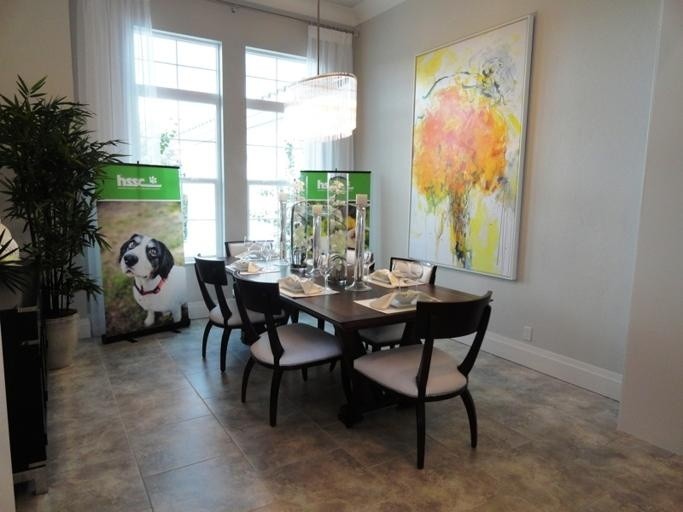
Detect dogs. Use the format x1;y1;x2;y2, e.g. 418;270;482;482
117;234;188;327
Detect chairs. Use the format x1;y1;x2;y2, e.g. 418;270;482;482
351;291;494;471
194;253;287;371
225;241;299;323
233;270;350;428
359;258;437;354
317;248;372;330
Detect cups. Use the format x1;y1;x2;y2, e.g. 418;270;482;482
279;264;288;278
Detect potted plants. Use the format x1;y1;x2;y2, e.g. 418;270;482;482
0;74;132;368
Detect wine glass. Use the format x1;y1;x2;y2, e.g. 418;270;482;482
393;260;424;299
261;243;274;272
244;235;254;252
316;256;335;293
362;251;375;278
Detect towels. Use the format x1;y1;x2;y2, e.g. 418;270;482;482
367;269;398;285
369;291;437;309
276;275;314;294
229;261;261;273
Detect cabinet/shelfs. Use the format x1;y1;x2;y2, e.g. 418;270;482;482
0;312;48;473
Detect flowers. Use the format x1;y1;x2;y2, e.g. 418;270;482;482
292;178;305;246
329;181;345;255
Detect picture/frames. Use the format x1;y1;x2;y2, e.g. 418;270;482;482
408;14;535;282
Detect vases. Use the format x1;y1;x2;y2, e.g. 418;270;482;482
289;175;308;270
327;173;348;285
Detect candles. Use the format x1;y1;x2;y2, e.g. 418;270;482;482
356;194;367;205
312;205;322;216
278;192;288;201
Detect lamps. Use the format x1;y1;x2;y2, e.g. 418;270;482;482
285;0;357;142
